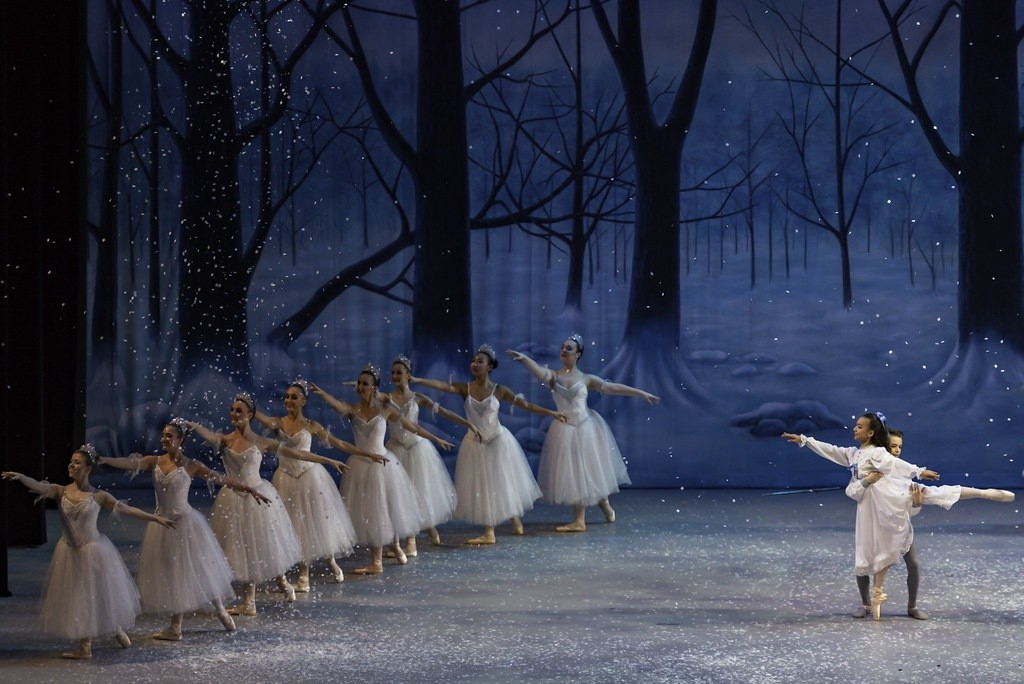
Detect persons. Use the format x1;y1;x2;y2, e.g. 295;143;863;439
343;354;483;545
1;443;176;659
507;333;661;531
781;412;1015;622
252;376;390;592
845;428;928;620
183;390;350;616
308;362;456;575
409;344;569;543
96;416;272;641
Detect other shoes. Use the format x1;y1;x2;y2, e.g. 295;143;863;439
467;534;496;544
512;516;524;535
387;548;405;558
872;602;880;621
853;605;871;617
332;563;344;583
114;625;130;649
215;606;235;631
389;542;408;565
277;579;297;603
62;647;92;659
428;527;440;545
605;509;615;524
986;488;1015;502
908;608;929;620
354;567;383;574
225;603;256;615
404;539;418;556
556;524;587;531
279;576;310;593
153;630;183;641
